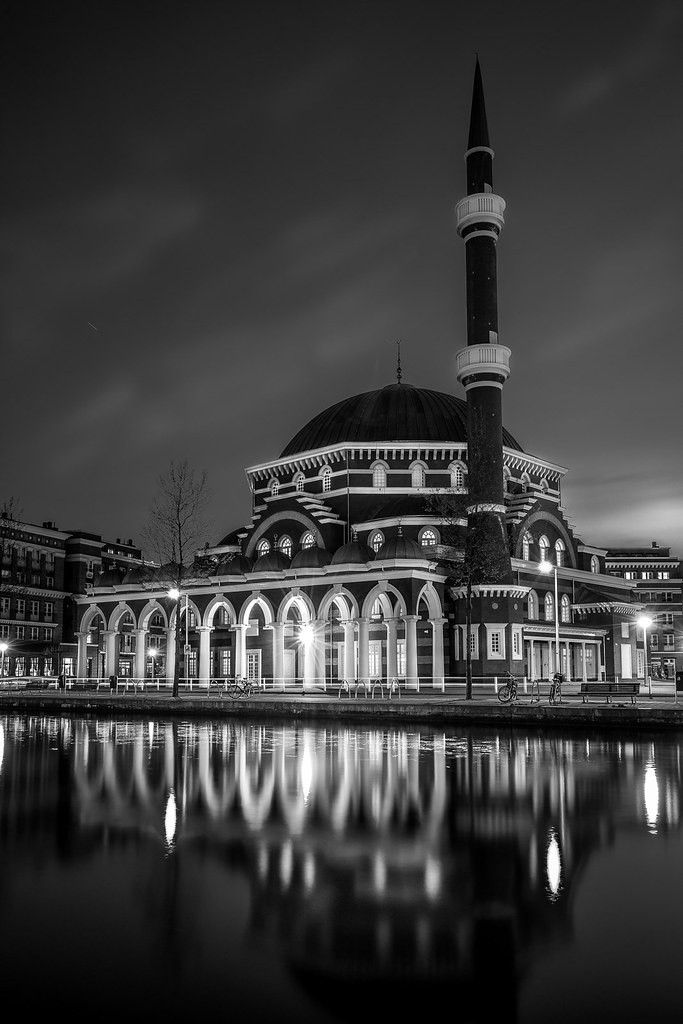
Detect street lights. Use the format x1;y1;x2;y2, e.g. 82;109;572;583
0;643;9;677
168;588;191;691
539;560;563;682
147;649;157;683
638;616;653;686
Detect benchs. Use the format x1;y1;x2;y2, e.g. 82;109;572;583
26;682;49;693
73;683;97;692
577;683;640;704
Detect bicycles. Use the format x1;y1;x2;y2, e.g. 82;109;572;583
548;672;561;706
227;674;255;700
497;671;520;702
